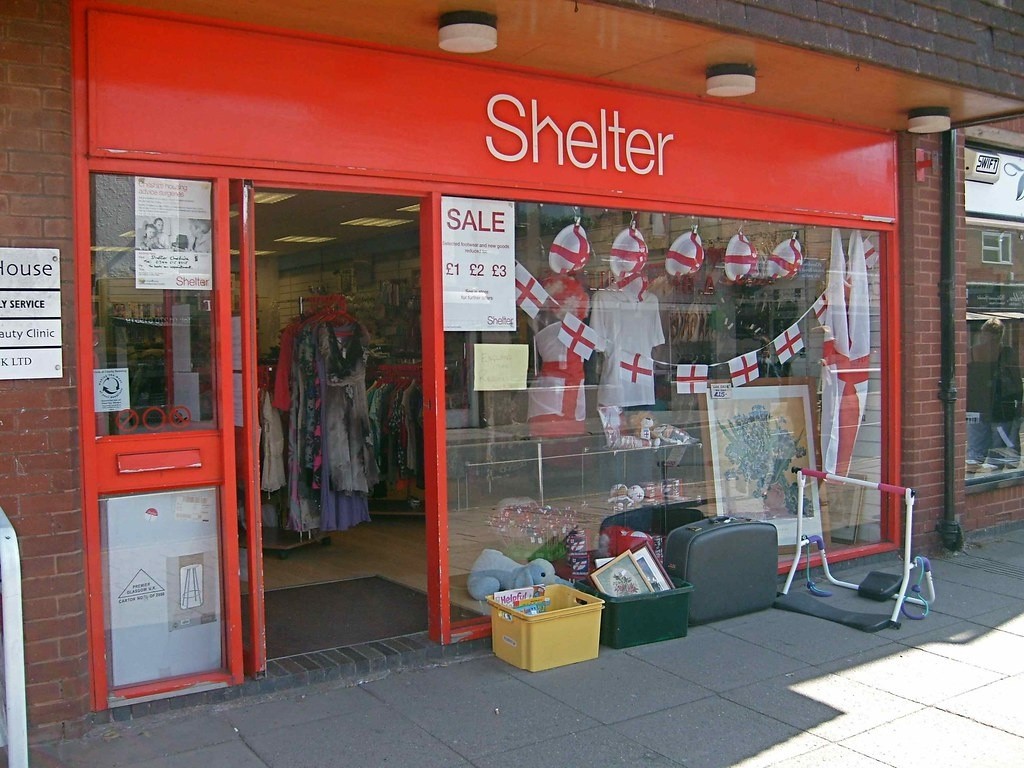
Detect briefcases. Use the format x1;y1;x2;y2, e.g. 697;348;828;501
598;504;704;554
663;515;778;626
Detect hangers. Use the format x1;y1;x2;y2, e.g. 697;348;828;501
287;294;359;336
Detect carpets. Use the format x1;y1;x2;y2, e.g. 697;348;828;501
240;574;485;663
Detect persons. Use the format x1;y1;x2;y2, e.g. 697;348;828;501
590;271;665;411
527;274;589;436
139;218;172;250
186;218;211;253
965;318;1023;476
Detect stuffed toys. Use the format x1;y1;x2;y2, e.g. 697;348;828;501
607;484;634;507
628;411;661;446
466;549;575;601
599;526;654;557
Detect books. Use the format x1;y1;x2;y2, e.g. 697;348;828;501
493;583;547;616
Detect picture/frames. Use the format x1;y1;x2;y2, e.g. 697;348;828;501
631;540;675;592
697;376;832;555
590;549;655;597
828;473;867;545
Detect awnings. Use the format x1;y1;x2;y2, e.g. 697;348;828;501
966;312;1024;321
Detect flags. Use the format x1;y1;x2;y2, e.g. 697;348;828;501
813;292;828;326
619;349;654;384
729;352;759;388
515;264;549;319
557;312;600;360
774;324;804;365
863;240;879;270
676;366;708;394
820;228;869;484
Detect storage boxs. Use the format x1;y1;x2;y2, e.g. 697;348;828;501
484;584;605;672
573;577;694;649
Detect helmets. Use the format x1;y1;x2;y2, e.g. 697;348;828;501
548;224;590;273
664;231;705;276
610;227;649;278
766;239;804;279
724;235;759;281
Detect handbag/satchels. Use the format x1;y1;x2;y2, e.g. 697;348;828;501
992;344;1022;422
